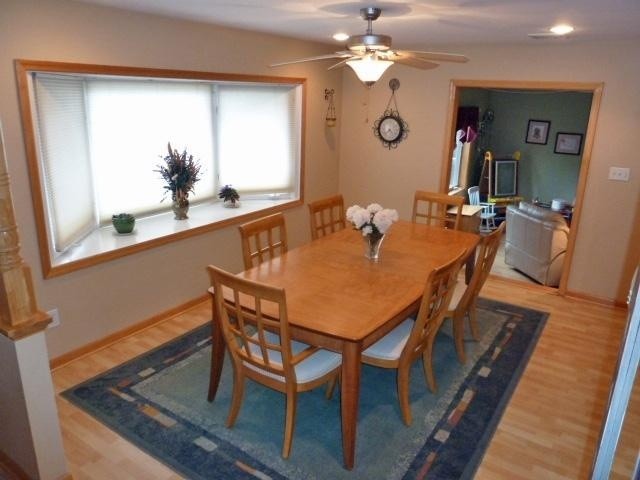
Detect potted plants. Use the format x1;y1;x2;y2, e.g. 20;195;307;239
218;184;241;208
156;142;201;220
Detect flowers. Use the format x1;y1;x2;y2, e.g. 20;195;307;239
346;203;398;263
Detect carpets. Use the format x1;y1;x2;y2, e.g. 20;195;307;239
56;297;553;480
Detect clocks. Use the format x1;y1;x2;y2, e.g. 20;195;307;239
372;77;409;149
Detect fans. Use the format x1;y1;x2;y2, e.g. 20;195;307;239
269;7;471;71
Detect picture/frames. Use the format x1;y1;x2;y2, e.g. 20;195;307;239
522;118;552;146
554;130;582;156
493;158;519;200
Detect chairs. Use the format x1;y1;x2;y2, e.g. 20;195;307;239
411;188;465;232
467;185;497;232
237;213;291;273
204;264;346;461
327;248;467;426
421;220;508;370
503;196;571;288
306;194;346;240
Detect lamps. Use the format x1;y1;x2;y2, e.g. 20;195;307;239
343;55;396;87
323;89;339;127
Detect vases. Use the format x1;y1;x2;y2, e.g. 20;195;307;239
361;233;387;262
111;212;136;234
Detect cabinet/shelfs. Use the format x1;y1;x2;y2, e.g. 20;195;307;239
444;204;482;234
